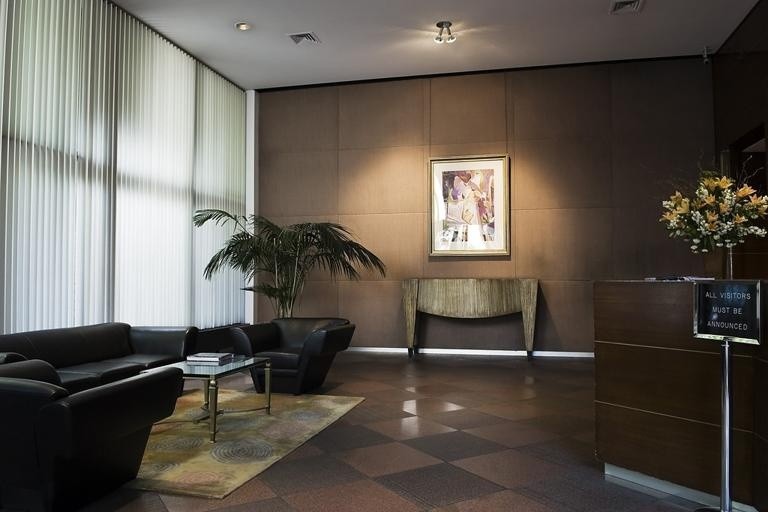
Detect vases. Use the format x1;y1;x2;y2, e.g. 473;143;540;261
720;246;735;280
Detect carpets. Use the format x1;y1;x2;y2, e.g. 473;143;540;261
121;385;366;500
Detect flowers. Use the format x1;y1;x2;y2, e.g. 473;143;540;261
654;145;768;256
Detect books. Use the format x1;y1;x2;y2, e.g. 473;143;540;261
186;352;232;361
186;359;233;366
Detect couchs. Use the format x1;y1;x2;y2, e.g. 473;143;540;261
0;322;198;389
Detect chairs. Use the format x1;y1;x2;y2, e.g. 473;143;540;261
230;316;355;396
0;360;184;512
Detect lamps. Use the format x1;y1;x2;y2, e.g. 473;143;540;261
430;22;457;44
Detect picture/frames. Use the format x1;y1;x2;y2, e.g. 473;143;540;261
427;153;511;257
